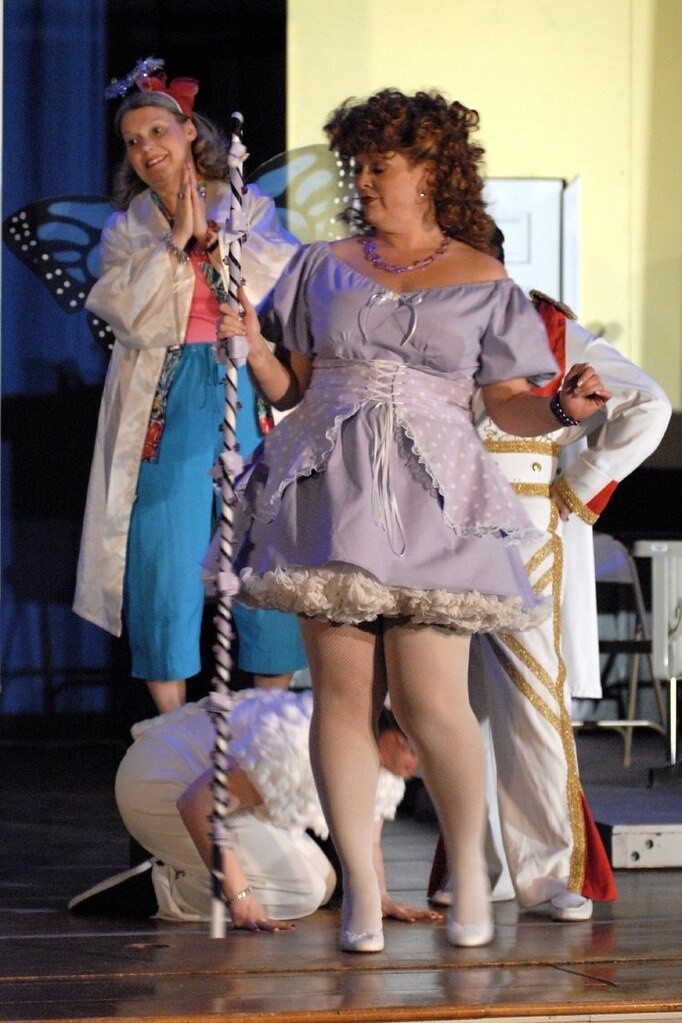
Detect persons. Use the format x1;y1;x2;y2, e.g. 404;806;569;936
67;54;308;710
57;687;422;931
424;279;673;918
197;90;611;957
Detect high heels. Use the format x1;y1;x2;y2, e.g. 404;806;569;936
341;895;383;952
446;872;495;947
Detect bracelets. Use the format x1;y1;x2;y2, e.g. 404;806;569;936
221;886;256;906
550;392;583;428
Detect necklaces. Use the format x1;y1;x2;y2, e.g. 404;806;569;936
360;230;456;277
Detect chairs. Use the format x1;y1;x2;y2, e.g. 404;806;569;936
572;534;668;768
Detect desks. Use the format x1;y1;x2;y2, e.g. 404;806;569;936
633;541;682;764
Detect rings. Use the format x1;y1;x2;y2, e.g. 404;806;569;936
175;189;186;201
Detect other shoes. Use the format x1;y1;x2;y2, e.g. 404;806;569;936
70;857;160;921
432;889;451;904
551;892;593;921
